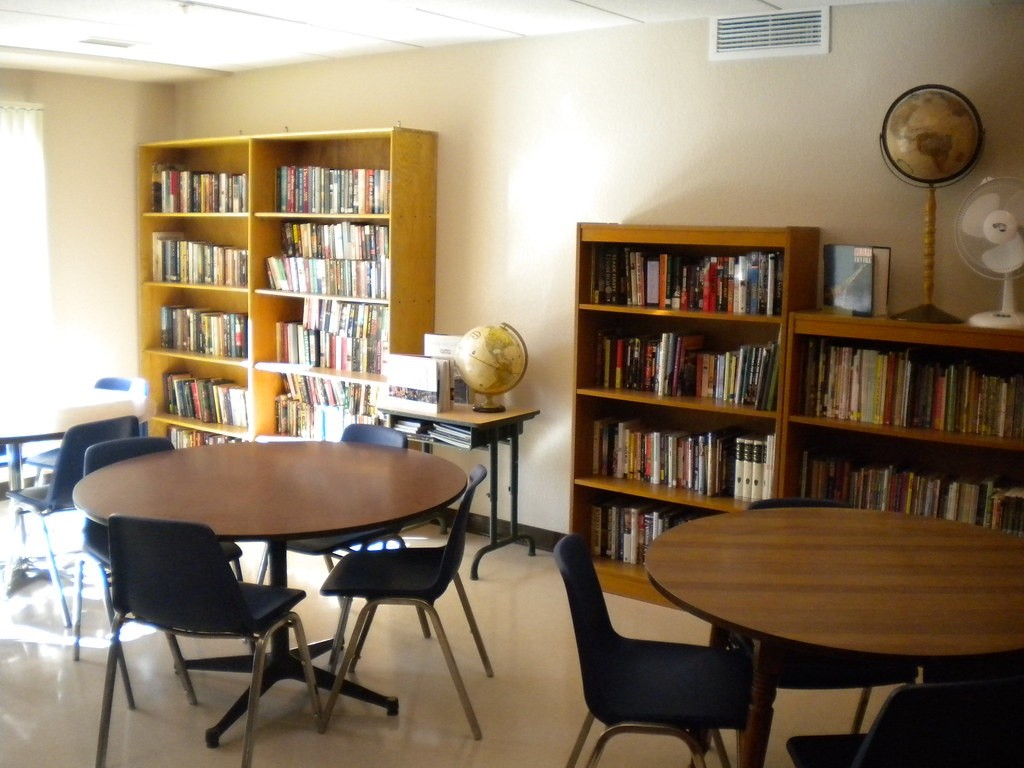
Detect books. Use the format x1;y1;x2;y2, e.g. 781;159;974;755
800;336;1024;446
586;489;709;571
154;302;250;365
587;401;779;504
258;220;389;304
148;155;250;216
274;297;390;380
150;224;249;293
584;239;784;319
274;366;383;447
821;241;893;317
792;436;1024;537
161;369;251;453
272;160;394;215
582;324;784;416
377;327;478;450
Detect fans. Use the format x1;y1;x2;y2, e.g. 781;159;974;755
953;177;1024;328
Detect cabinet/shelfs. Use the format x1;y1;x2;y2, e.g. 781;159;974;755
136;127;438;450
569;226;820;610
778;313;1024;543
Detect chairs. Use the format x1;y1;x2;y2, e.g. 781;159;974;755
256;424;433;639
553;533;751;768
321;464;493;741
6;416;141;627
731;497;919;734
96;514;323;768
784;682;1023;768
25;376;151;487
72;435;257;709
1;443;27;489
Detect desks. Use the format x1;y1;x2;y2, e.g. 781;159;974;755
643;508;1023;768
0;388;158;599
71;441;468;748
377;405;540;580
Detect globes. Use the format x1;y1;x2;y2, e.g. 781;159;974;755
878;82;986;325
454;322;529;412
